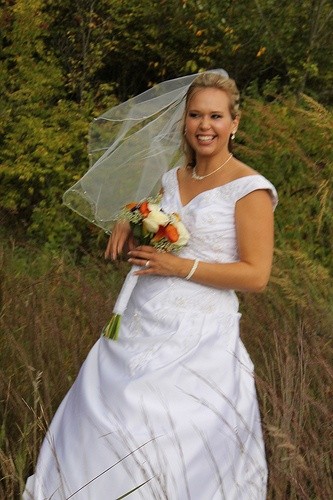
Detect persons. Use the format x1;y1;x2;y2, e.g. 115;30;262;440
23;69;279;500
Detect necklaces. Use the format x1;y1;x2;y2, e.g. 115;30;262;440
192;150;233;179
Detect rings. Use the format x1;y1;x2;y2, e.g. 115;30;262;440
145;260;150;268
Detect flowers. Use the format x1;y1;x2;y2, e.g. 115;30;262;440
101;192;191;342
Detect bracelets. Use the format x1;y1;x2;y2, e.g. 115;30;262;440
182;258;200;281
